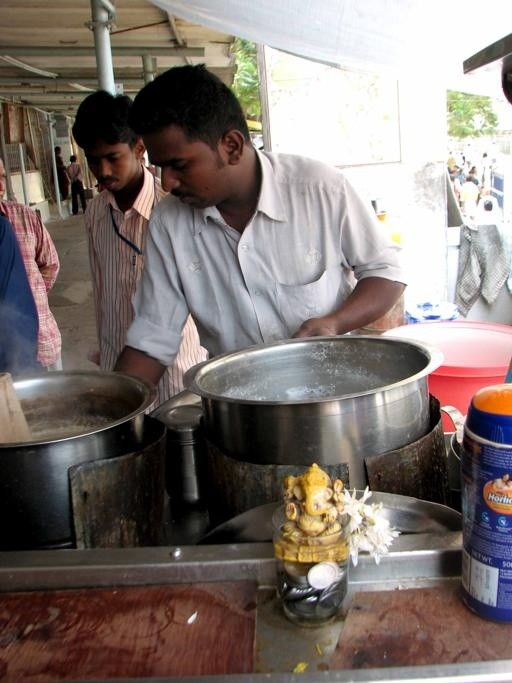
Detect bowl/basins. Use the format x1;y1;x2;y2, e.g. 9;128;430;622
379;317;512;431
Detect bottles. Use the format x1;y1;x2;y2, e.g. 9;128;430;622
456;380;512;629
157;403;210;507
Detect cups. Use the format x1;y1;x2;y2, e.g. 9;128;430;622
271;504;352;628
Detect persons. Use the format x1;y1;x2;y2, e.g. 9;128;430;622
447;148;501;227
0;158;62;384
114;65;405;393
64;155;86;215
51;146;70;200
72;91;208;419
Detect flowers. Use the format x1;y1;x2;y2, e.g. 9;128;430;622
343;487;401;568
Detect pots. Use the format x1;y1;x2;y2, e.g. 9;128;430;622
179;330;444;494
1;368;165;548
